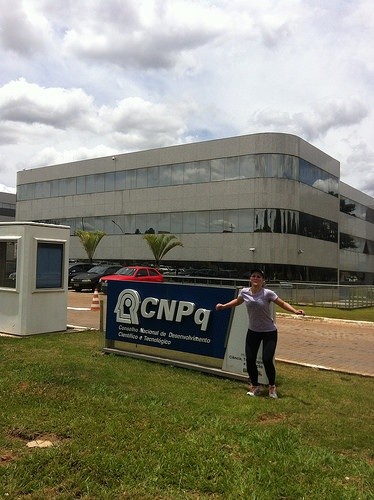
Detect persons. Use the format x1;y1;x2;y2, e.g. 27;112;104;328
215;267;306;398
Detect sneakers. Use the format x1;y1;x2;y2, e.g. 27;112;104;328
246;386;261;396
268;387;278;399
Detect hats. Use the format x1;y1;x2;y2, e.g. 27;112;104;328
251;267;265;278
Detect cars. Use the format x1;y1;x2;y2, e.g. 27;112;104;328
98;266;163;292
71;264;123;292
68;262;95;287
